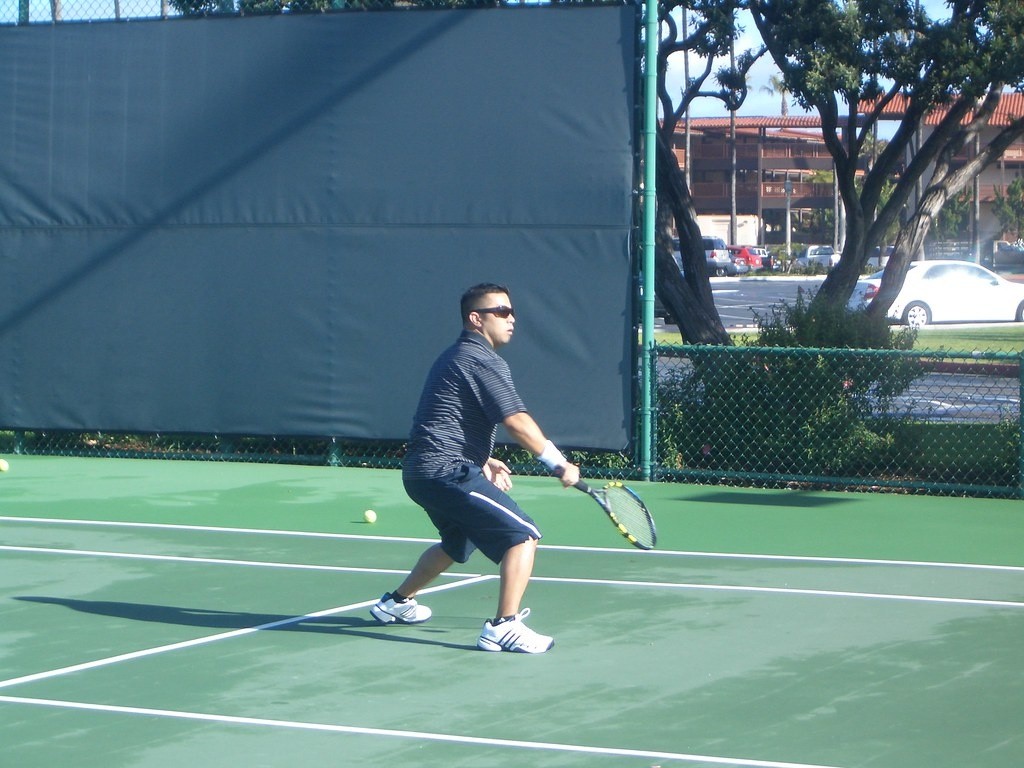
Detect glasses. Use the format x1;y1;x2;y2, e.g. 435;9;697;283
470;306;515;318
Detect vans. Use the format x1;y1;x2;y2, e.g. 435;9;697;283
671;235;732;271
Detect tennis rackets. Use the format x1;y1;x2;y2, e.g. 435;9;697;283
536;457;657;549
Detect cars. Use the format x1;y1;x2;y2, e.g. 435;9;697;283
844;260;1024;326
727;245;841;278
715;251;749;277
866;245;895;273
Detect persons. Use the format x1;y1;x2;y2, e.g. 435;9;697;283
368;284;580;654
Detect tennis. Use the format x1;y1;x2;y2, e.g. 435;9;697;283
364;510;377;522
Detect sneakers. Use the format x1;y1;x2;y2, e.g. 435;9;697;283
477;607;554;653
370;592;432;624
